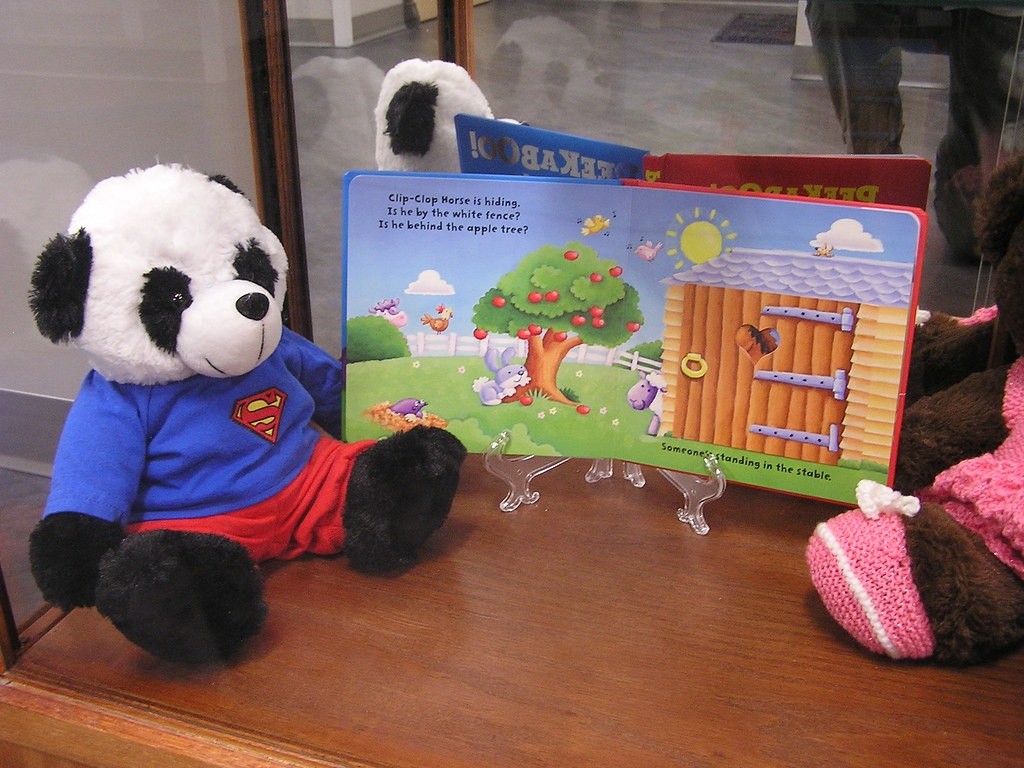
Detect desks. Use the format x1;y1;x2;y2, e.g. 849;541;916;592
0;450;1023;768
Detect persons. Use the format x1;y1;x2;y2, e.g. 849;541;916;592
805;0;1024;263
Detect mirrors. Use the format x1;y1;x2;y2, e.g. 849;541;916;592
237;0;1024;409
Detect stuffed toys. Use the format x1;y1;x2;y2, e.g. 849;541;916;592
905;152;1024;408
26;162;469;662
807;219;1024;665
374;58;529;172
